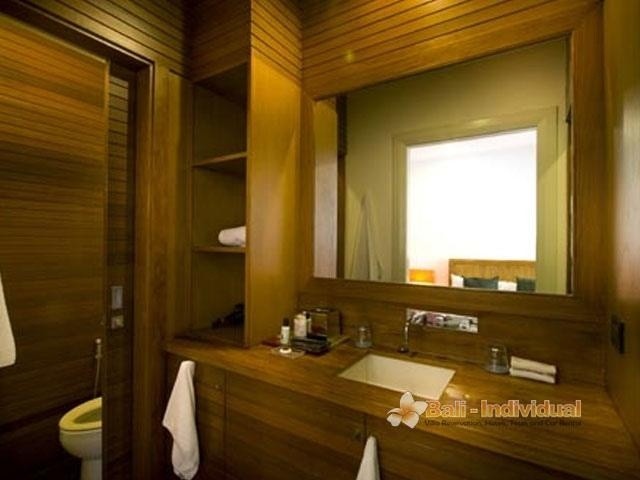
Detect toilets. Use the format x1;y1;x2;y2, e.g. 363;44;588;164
57;396;102;480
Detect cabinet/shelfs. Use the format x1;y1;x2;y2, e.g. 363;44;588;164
177;56;300;351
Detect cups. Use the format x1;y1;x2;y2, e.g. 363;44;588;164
355;325;372;349
485;344;509;373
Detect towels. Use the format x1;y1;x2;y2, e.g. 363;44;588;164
0;274;17;369
508;355;557;385
355;435;381;480
217;225;247;247
161;359;201;480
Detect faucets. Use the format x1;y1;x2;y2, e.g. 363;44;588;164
395;320;411;353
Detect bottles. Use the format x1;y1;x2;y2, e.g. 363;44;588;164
294;314;307;337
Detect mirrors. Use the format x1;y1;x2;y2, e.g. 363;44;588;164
311;30;577;298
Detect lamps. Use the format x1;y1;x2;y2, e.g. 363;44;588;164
406;268;436;286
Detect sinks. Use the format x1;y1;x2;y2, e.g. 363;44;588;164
336;349;457;401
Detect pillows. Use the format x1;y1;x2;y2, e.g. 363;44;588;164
449;273;537;292
